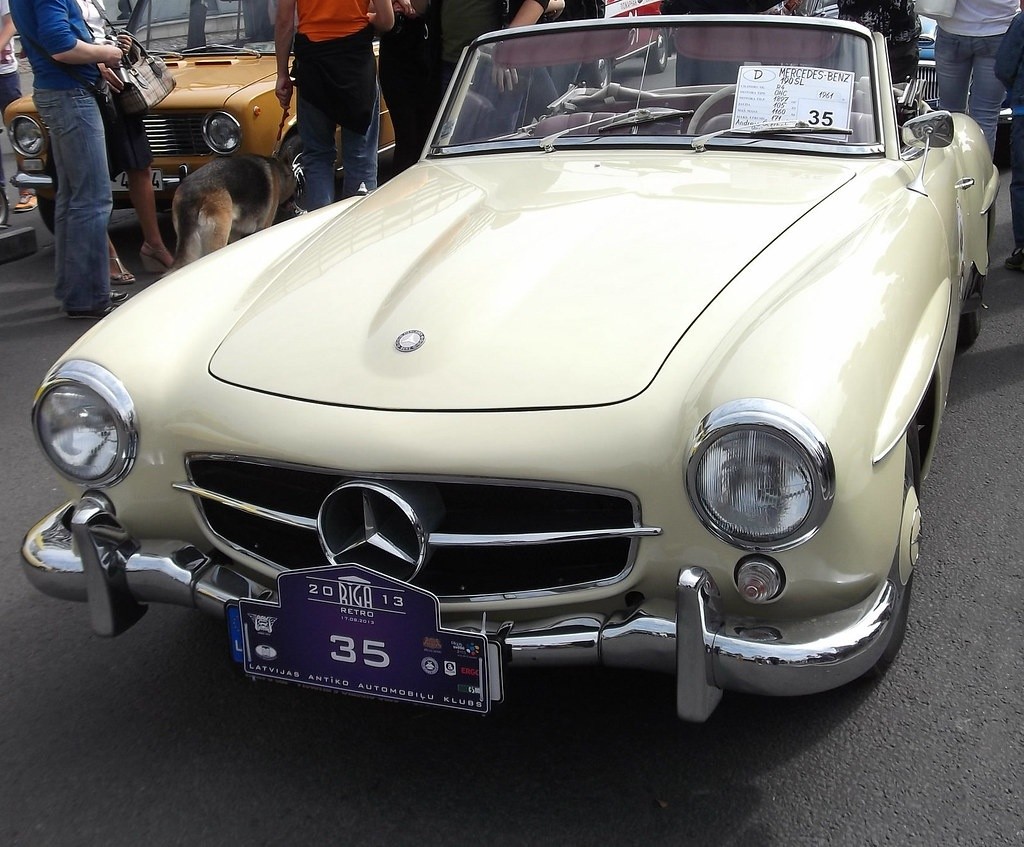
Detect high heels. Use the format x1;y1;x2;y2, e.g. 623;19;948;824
139;241;175;273
109;258;137;285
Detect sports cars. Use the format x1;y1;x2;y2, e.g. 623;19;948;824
21;17;999;724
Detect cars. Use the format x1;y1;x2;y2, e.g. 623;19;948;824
582;0;1024;124
530;0;670;93
811;1;940;109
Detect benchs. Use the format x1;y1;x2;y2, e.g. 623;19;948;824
543;89;923;127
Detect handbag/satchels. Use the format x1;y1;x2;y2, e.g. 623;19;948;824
913;0;957;21
113;34;177;119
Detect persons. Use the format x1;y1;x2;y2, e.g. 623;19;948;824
660;0;1024;269
276;0;607;212
0;0;174;317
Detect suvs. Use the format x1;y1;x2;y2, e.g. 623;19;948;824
4;0;397;237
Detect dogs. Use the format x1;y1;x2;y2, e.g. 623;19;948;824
147;145;297;284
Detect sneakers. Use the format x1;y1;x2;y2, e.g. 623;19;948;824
1004;248;1024;270
13;192;38;212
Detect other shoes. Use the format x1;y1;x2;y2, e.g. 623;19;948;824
109;289;129;301
65;303;119;319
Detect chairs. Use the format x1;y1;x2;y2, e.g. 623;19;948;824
698;107;881;145
533;111;672;139
